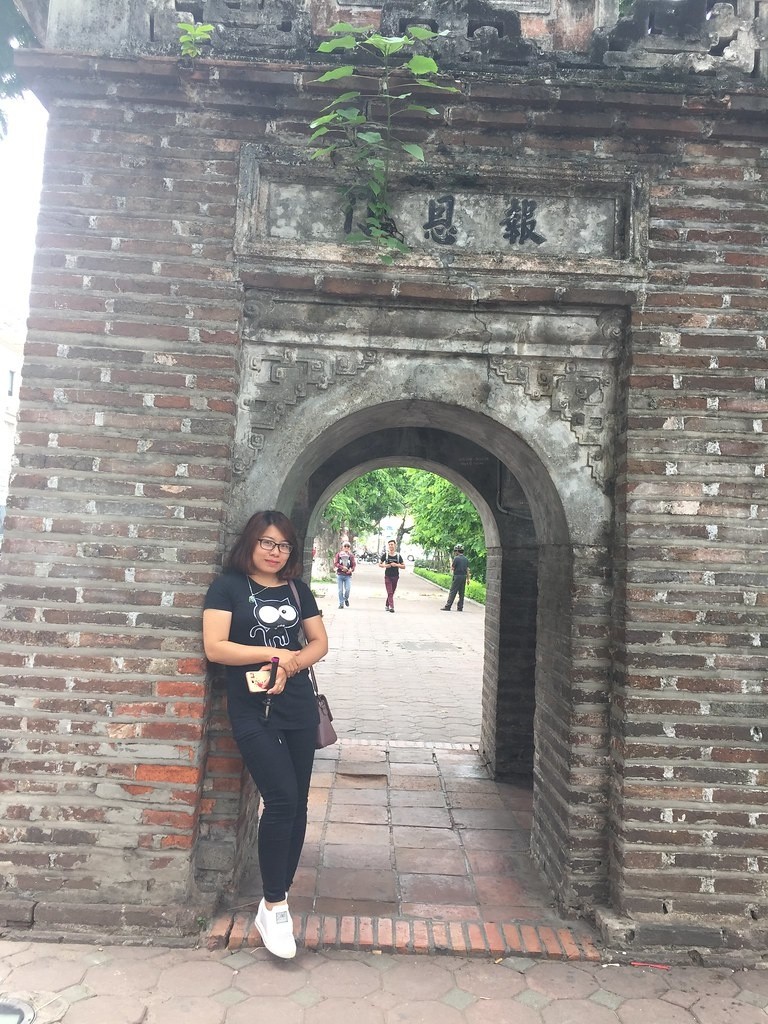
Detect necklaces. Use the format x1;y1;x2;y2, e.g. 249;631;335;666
246;571;277;603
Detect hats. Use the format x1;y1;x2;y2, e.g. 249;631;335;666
454;544;464;550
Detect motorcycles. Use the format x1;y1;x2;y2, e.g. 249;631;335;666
357;549;378;564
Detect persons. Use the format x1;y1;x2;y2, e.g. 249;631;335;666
379;540;405;612
334;542;356;608
441;544;470;611
203;511;328;958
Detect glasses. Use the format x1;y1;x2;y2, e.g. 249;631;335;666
257;538;294;554
344;546;350;548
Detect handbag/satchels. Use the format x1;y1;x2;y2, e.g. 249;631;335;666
314;694;337;749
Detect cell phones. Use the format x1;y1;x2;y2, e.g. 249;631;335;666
245;671;271;692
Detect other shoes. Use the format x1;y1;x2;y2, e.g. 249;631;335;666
389;609;394;612
345;601;349;606
440;608;450;611
457;608;462;611
339;605;344;608
385;607;389;611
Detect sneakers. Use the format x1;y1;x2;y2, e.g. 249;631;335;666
254;892;296;958
